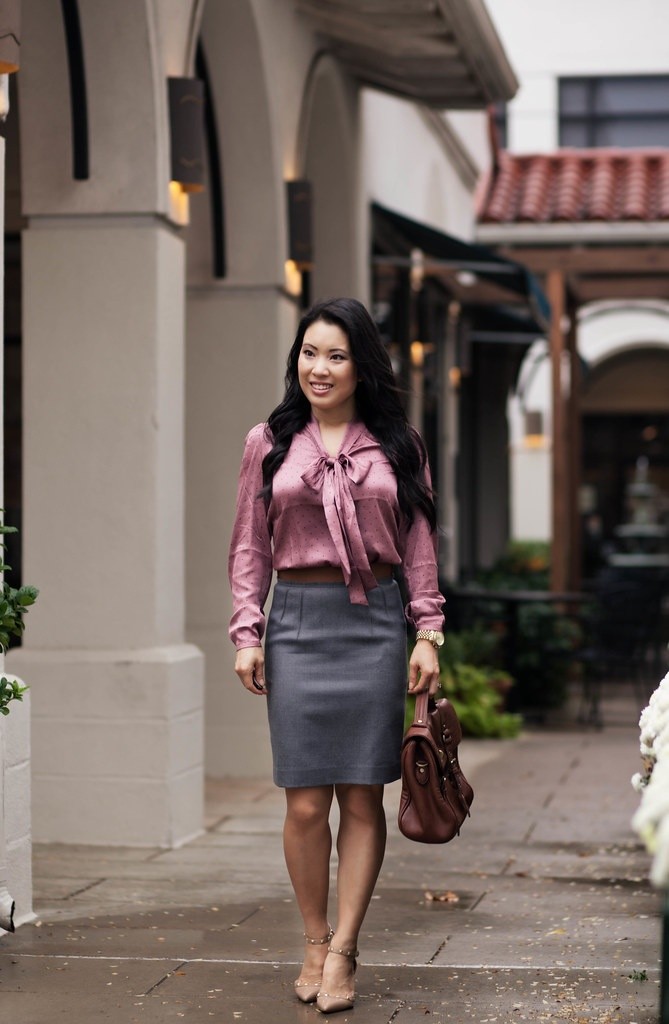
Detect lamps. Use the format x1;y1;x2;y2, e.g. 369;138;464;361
169;75;206;194
523;407;545;445
286;178;316;265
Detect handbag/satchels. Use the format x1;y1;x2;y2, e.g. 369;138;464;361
399;690;474;843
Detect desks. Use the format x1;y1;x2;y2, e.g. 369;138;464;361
454;590;598;716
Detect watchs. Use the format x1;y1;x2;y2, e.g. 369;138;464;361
414;629;445;650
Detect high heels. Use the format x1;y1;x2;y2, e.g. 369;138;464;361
318;947;359;1013
294;924;334;1002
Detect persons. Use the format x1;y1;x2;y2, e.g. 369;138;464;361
225;298;450;1016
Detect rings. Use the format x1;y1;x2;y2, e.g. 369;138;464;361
430;682;442;690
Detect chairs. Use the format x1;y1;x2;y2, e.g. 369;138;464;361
539;563;668;723
